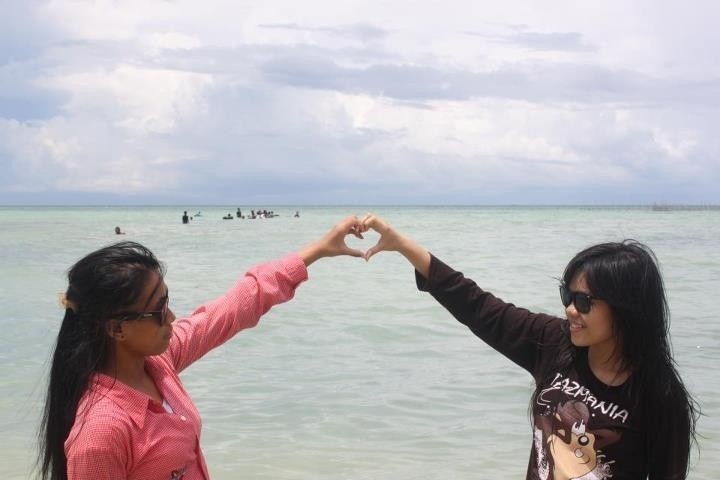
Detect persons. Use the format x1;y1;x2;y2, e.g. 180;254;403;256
55;208;365;480
358;214;691;480
179;204;282;226
115;227;121;235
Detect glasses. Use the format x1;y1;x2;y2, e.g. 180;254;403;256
104;290;168;325
560;286;608;314
